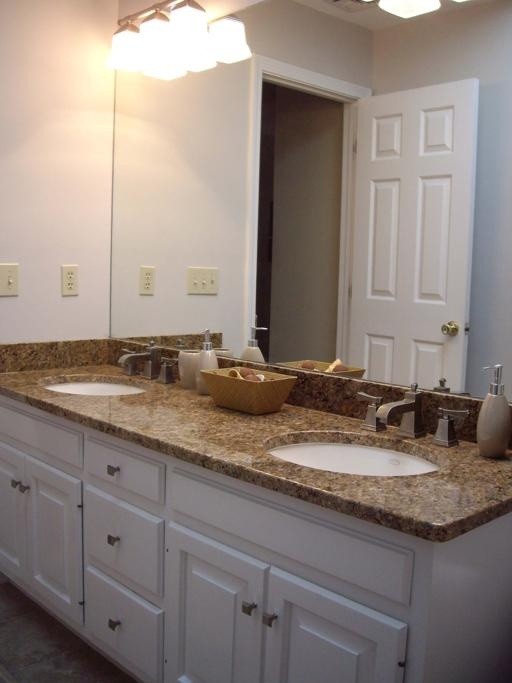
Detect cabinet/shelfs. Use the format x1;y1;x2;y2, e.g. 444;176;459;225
165;394;510;682
0;395;82;636
80;395;165;683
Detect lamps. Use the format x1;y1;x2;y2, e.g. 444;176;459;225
109;0;209;49
142;15;251;81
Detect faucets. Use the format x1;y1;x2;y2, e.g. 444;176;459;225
434;377;472;397
118;341;163;380
375;382;427;439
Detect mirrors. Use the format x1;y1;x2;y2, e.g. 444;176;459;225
105;2;512;411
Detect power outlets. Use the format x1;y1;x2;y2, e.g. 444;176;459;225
140;268;155;296
60;265;80;298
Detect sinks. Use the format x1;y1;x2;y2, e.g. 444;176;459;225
33;371;151;398
256;429;446;479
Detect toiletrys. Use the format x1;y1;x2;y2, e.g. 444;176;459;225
240;326;269;364
476;364;511;456
196;329;221;396
